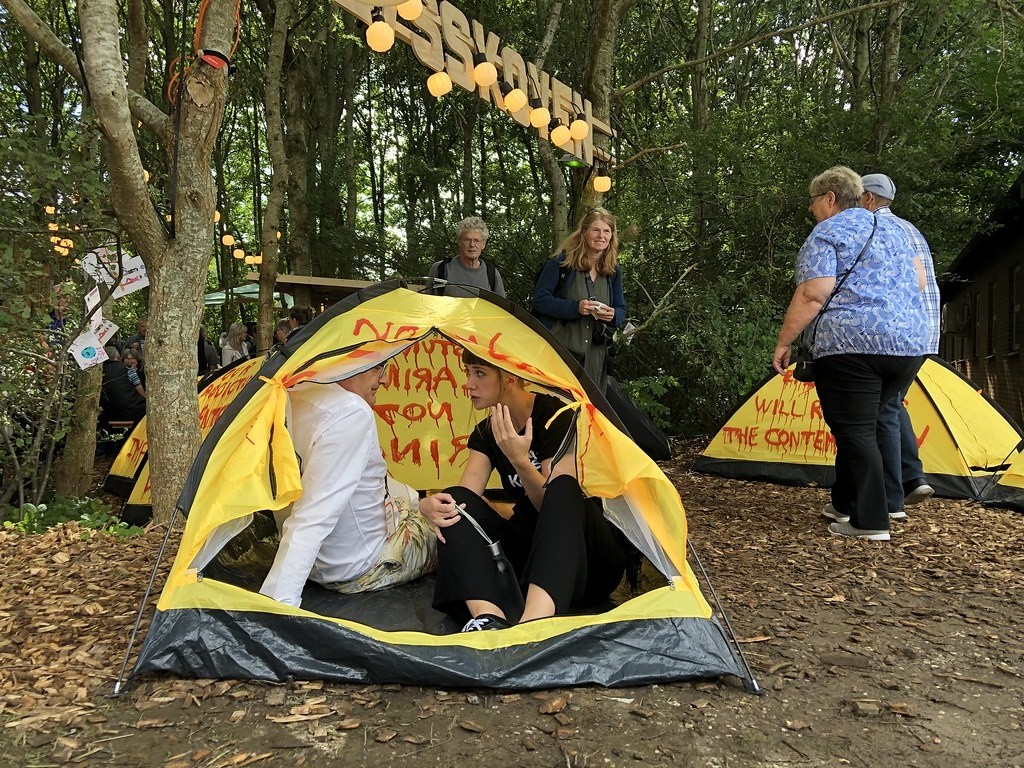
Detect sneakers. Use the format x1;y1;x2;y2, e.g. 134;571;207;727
902;477;935;504
828;522;890;541
887;507;908;519
823;503;850;523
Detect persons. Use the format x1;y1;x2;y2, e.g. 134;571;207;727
96;304;316;457
531;207;626;399
419;348;627;633
773;164;940;540
424;216;506;298
46;285;71;352
259;361;516;609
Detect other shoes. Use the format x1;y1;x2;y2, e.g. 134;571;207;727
462;614;512;632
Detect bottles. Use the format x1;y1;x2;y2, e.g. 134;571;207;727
486;540;525;626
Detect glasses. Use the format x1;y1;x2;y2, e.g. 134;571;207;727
808;192;827;203
243;331;248;333
123;356;137;362
377;362;389;378
276;328;283;331
287;318;294;321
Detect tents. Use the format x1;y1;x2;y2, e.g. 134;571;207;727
93;342;285;529
684;354;1024;516
204;283;295;310
108;275;765;700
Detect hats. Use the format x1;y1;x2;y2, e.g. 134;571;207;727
126;335;140;346
860;174;896;200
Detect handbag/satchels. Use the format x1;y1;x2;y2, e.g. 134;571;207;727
591;319;616;345
793;346;816;382
